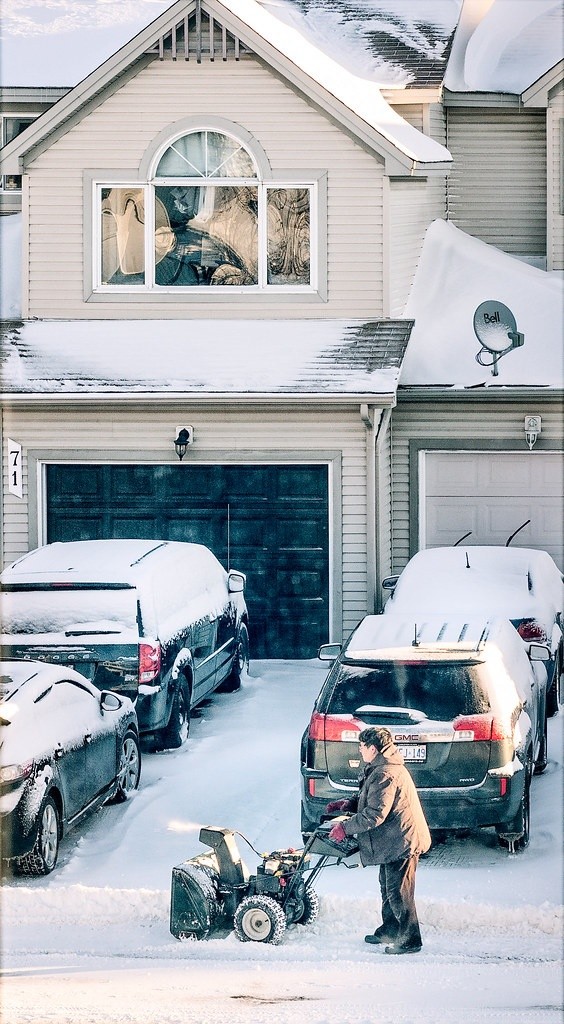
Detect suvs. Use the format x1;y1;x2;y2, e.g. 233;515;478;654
379;547;564;720
298;612;552;856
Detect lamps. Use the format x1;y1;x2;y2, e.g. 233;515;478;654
173;425;195;462
525;415;542;451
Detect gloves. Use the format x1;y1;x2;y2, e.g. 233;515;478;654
327;799;350;813
328;822;345;844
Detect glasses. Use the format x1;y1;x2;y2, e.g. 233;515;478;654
359;744;366;750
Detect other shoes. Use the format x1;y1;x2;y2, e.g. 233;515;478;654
365;935;394;944
385;943;421;954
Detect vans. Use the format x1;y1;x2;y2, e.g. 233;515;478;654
1;538;251;753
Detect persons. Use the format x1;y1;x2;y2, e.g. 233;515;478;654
324;726;433;954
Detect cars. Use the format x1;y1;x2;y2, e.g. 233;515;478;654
0;656;141;878
